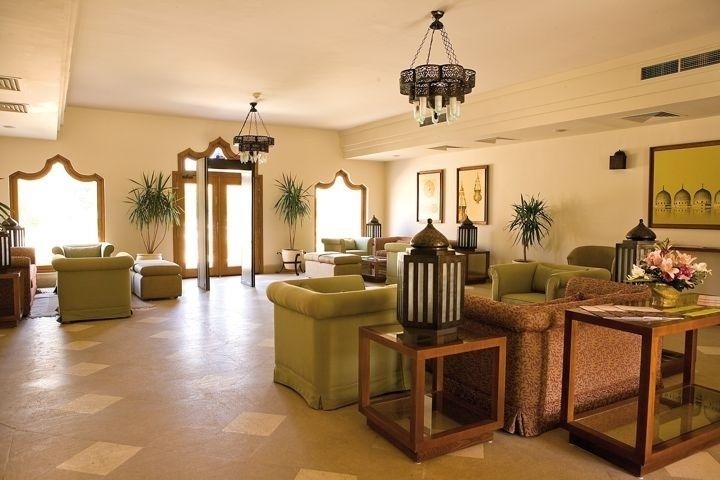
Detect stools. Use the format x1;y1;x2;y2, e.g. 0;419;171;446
130;261;139;292
134;260;182;299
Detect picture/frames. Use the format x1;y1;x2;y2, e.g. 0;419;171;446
646;139;719;228
415;165;489;225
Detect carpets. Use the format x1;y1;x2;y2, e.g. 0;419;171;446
29;284;157;318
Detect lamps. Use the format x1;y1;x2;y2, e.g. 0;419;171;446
232;102;275;164
615;220;657;285
394;218;466;347
366;214;382;238
0;227;12;273
399;10;475;126
3;215;25;248
457;216;478;253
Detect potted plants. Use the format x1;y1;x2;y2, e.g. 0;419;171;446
503;192;553;264
123;170;186;260
274;170;316;270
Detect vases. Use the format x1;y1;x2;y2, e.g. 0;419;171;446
647;281;682;308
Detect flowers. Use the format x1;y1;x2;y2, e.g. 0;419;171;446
629;249;711;287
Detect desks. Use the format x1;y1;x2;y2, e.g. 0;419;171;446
358;306;720;476
0;267;24;328
457;250;491;285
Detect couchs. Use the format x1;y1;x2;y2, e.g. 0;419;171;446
384;242;411;286
52;242;135;324
371;234;459;257
9;246;38;315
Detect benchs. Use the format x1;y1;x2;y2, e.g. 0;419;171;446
303;251;363;276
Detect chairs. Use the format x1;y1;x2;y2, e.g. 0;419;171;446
321;237;374;256
490;245;615;304
266;275;402;411
